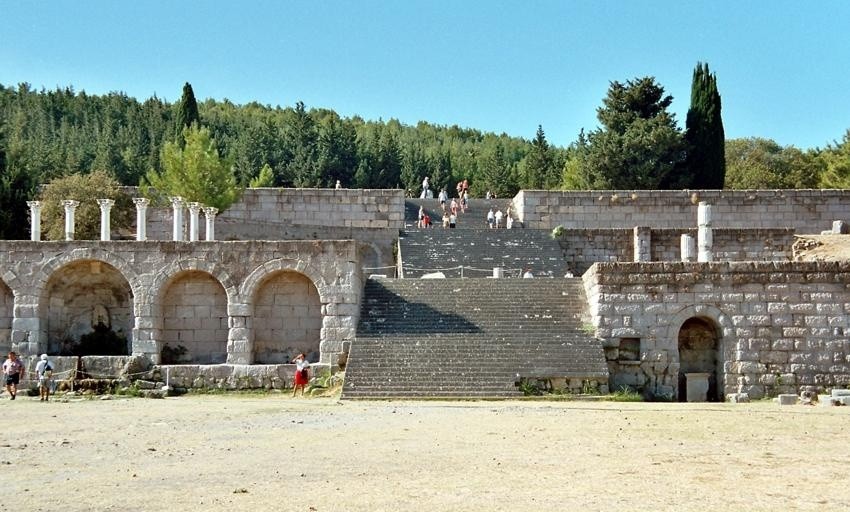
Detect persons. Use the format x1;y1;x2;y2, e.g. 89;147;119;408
1;352;26;401
335;179;343;189
33;352;57;403
415;175;514;231
290;354;310;397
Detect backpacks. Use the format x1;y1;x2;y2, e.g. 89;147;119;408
41;360;53;378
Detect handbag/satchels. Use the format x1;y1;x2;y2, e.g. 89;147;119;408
300;369;309;380
